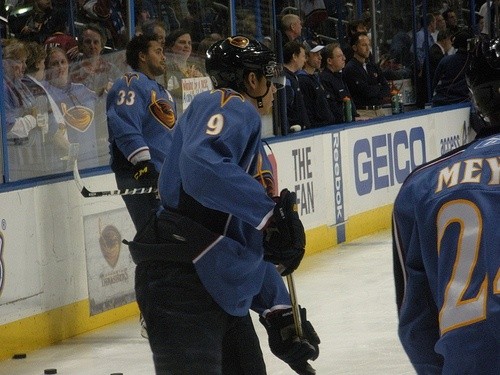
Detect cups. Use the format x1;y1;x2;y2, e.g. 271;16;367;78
36;95;48;127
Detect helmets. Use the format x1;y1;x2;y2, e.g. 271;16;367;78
205;34;282;88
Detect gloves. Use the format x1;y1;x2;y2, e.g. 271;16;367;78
131;160;162;207
259;303;320;375
264;188;306;276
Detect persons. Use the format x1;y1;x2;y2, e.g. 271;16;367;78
121;34;321;375
0;0;272;181
272;0;500;137
392;36;500;375
105;33;177;340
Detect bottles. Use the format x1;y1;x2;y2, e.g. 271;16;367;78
342;96;352;124
397;94;404;113
391;90;400;114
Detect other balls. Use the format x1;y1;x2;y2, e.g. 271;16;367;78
13;353;27;359
44;368;57;375
110;372;123;375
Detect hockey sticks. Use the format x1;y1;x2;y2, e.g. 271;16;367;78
73;158;158;199
286;272;304;339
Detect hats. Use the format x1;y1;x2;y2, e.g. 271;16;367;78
465;36;500;132
304;40;324;53
